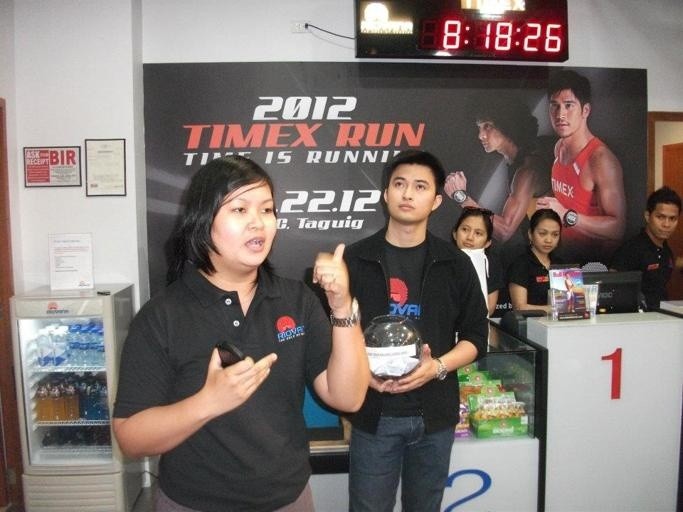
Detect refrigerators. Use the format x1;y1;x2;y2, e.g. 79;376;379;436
8;283;135;511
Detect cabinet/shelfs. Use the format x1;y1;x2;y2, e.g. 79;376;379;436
302;320;549;512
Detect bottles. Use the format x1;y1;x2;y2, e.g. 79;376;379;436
35;324;109;421
361;316;422;381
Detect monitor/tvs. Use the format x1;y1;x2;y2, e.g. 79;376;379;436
583;271;642;314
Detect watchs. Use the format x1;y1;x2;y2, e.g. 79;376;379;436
452;190;469;206
563;208;578;229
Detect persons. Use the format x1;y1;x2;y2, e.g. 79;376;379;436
615;184;682;312
536;69;627;263
338;154;491;511
443;93;551;274
504;208;571;317
112;155;370;512
448;208;507;316
